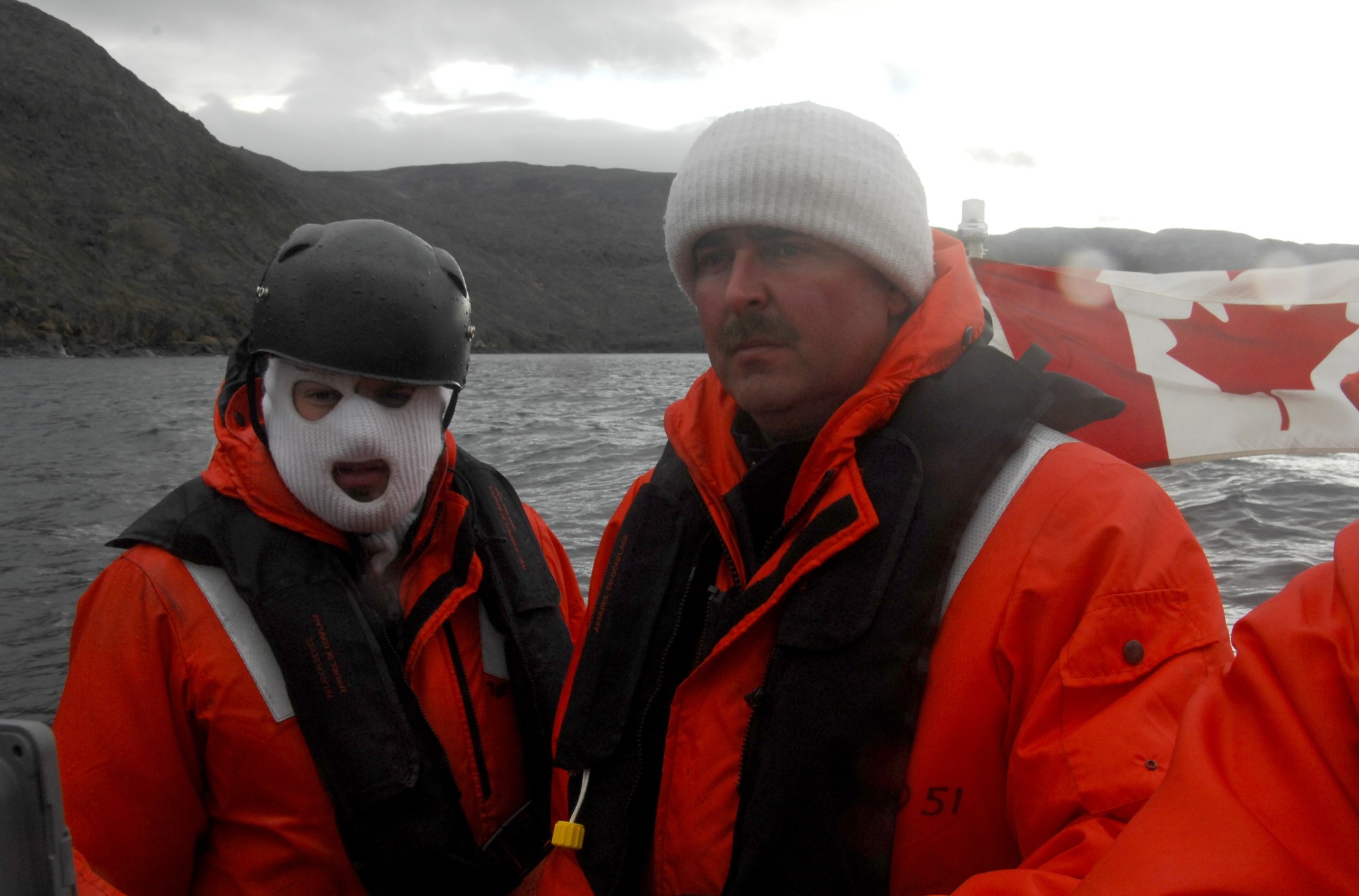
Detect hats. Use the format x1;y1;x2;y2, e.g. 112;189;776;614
667;99;936;306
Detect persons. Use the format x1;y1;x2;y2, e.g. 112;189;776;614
1075;515;1358;895
49;218;588;896
552;105;1232;896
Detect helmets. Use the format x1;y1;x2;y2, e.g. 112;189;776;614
249;218;473;392
